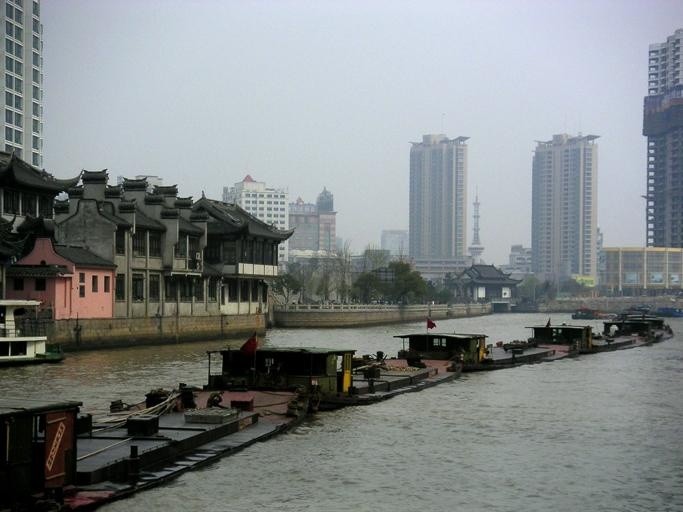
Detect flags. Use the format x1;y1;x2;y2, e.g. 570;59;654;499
427;317;437;331
239;332;257;355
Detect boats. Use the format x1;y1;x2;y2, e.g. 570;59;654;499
393;323;581;373
524;306;674;355
0;298;67;365
571;306;617;321
620;305;682;318
205;334;463;413
1;378;310;511
